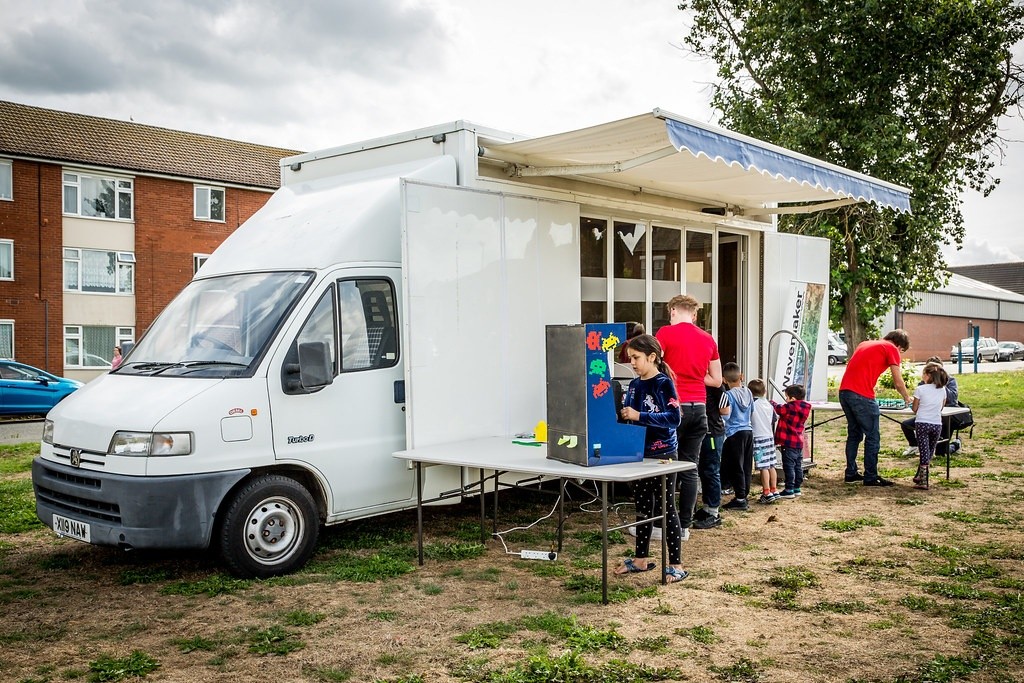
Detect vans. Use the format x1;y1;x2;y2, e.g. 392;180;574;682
828;331;849;366
950;336;1001;363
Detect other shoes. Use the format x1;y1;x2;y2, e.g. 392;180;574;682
903;446;917;455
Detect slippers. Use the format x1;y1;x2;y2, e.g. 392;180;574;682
619;559;655;574
665;567;689;582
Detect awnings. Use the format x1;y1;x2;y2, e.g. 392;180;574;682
496;106;913;215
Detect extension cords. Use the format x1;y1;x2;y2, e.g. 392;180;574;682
521;549;557;561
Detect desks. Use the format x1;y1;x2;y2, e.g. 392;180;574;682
805;401;970;486
392;437;696;605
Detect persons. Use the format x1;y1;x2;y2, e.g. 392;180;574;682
720;362;754;510
769;384;811;499
911;363;948;489
902;357;957;455
112;346;122;368
749;379;781;503
692;385;730;529
629;295;722;540
839;329;914;485
614;334;689;583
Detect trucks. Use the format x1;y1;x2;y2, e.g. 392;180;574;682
28;105;915;578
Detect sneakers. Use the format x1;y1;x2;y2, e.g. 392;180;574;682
863;476;893;487
845;472;864;483
693;512;722;528
794;489;801;495
680;527;689;540
692;508;710;521
721;497;747;509
779;489;796;498
628;525;662;540
756;492;775;503
773;491;781;499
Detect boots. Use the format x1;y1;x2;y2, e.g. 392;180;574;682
913;467;921;482
913;464;929;490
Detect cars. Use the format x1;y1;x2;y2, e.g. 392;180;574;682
997;342;1024;361
0;360;86;419
65;352;113;367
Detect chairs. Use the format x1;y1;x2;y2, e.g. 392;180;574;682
280;300;323;342
343;291;396;368
